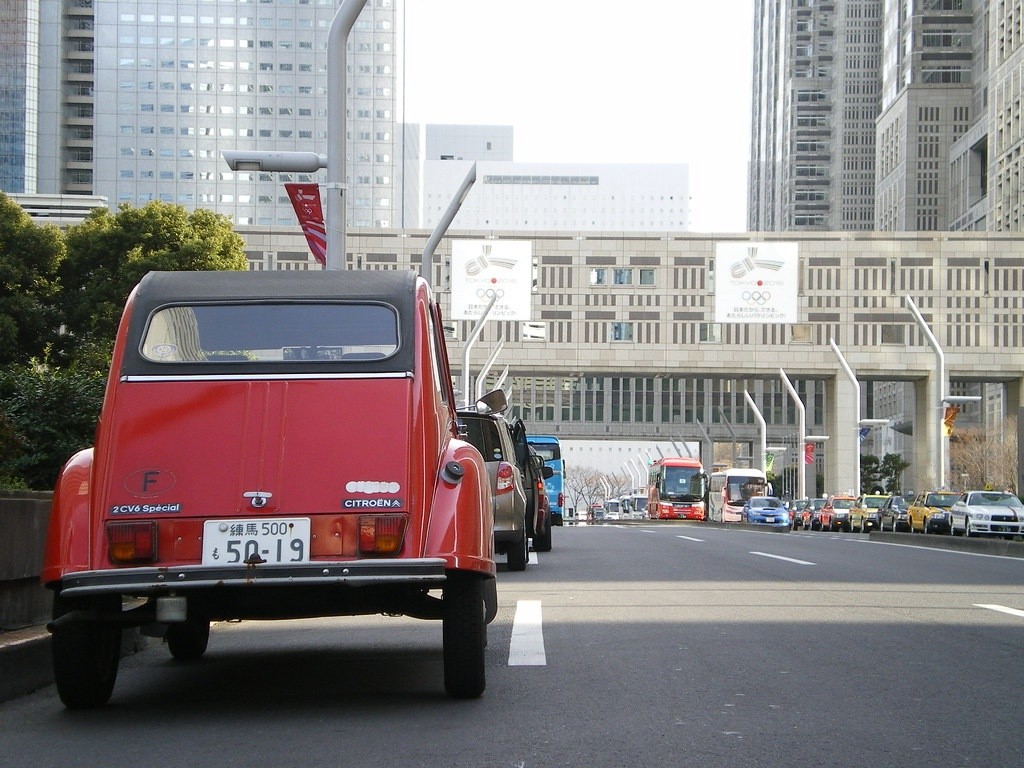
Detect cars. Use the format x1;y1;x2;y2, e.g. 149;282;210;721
46;269;498;709
907;485;960;535
741;489;853;533
948;482;1024;540
877;490;916;531
848;491;891;532
455;413;544;571
517;443;553;552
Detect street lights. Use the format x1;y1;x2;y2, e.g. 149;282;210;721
745;389;787;496
421;159;476;289
599;418;728;507
779;367;830;499
904;292;983;491
222;146;347;271
829;336;890;495
718;406;755;468
475;334;504;412
460;292;497;412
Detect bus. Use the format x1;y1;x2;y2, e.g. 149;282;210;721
527;435;566;527
587;458;773;521
